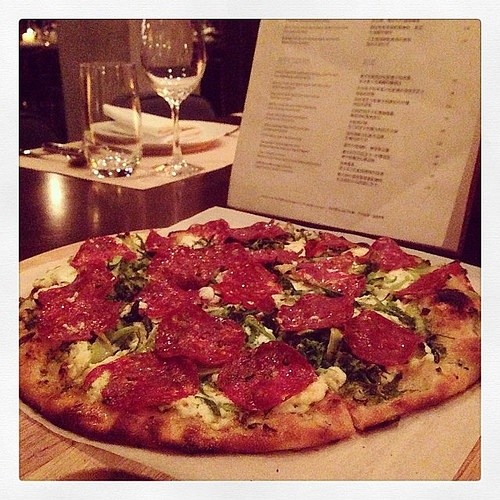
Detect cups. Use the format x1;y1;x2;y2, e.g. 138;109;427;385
80;62;142;178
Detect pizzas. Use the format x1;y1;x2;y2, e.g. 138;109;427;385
18;219;481;451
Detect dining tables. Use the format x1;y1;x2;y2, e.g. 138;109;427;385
20;113;480;479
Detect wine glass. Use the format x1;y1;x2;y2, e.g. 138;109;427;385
138;19;205;177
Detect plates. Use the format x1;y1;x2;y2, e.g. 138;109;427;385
86;120;239;153
